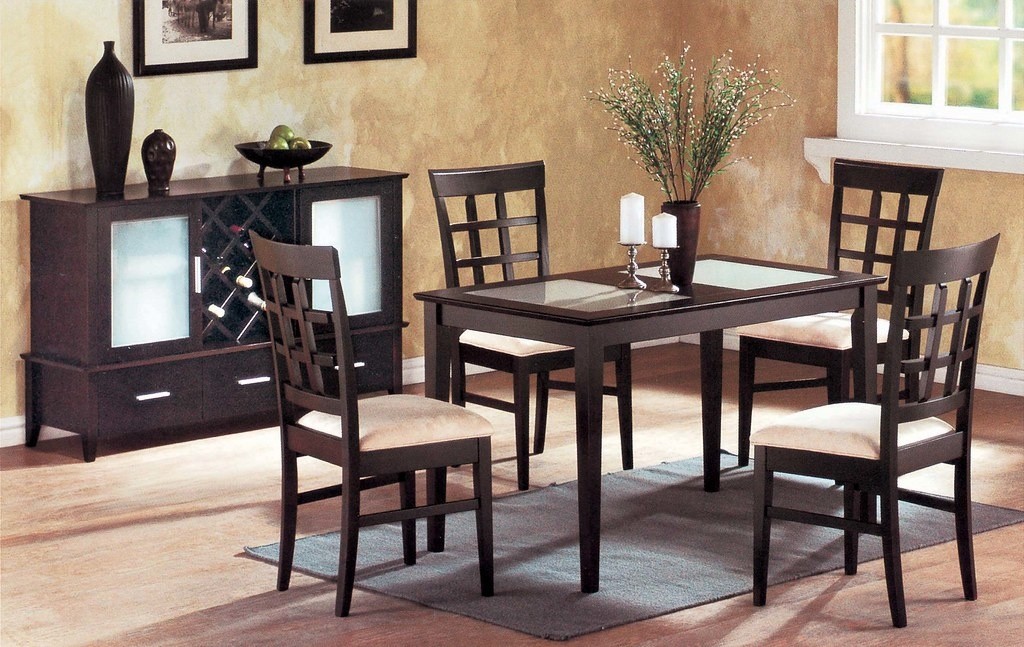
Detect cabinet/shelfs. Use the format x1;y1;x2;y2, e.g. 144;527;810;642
18;167;411;462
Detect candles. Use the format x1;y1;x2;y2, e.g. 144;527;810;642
620;193;644;245
651;212;678;246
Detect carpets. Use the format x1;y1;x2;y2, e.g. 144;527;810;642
245;450;1024;639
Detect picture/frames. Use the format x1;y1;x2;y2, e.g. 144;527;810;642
303;0;417;65
132;0;258;77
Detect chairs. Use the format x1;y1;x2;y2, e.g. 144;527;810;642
746;232;1000;628
428;159;634;490
738;158;945;485
247;229;493;618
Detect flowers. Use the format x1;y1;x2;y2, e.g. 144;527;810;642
579;38;797;202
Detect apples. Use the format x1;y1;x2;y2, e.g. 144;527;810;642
264;124;313;149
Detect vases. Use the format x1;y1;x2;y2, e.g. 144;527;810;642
141;129;177;192
85;41;134;195
659;200;699;285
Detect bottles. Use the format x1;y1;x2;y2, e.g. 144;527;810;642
85;40;135;201
141;129;176;197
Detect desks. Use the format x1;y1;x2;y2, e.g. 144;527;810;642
414;254;889;594
